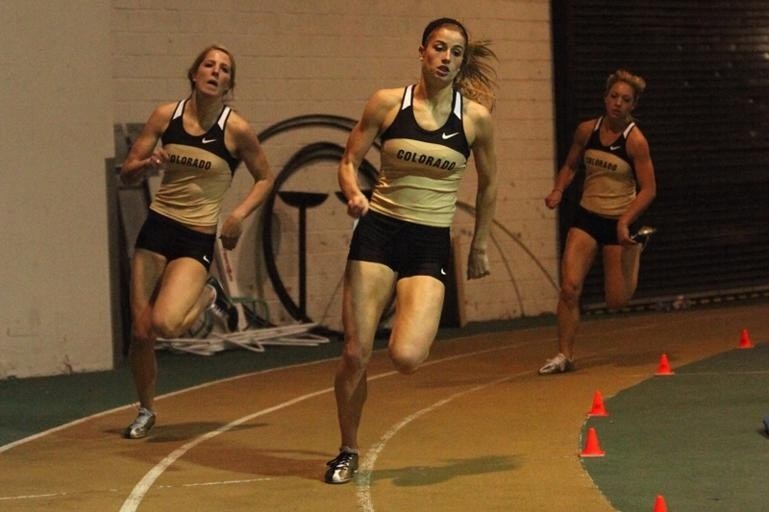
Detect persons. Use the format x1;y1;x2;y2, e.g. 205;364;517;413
537;69;657;374
324;18;500;484
119;43;275;438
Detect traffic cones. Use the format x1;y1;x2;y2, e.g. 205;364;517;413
650;494;669;512
734;327;757;350
581;388;611;417
652;351;677;377
574;427;607;459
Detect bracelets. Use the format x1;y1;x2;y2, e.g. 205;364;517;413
552;189;563;194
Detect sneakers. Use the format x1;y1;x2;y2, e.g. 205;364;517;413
204;277;238;333
324;446;359;483
631;227;656;247
123;408;156;440
538;352;574;375
